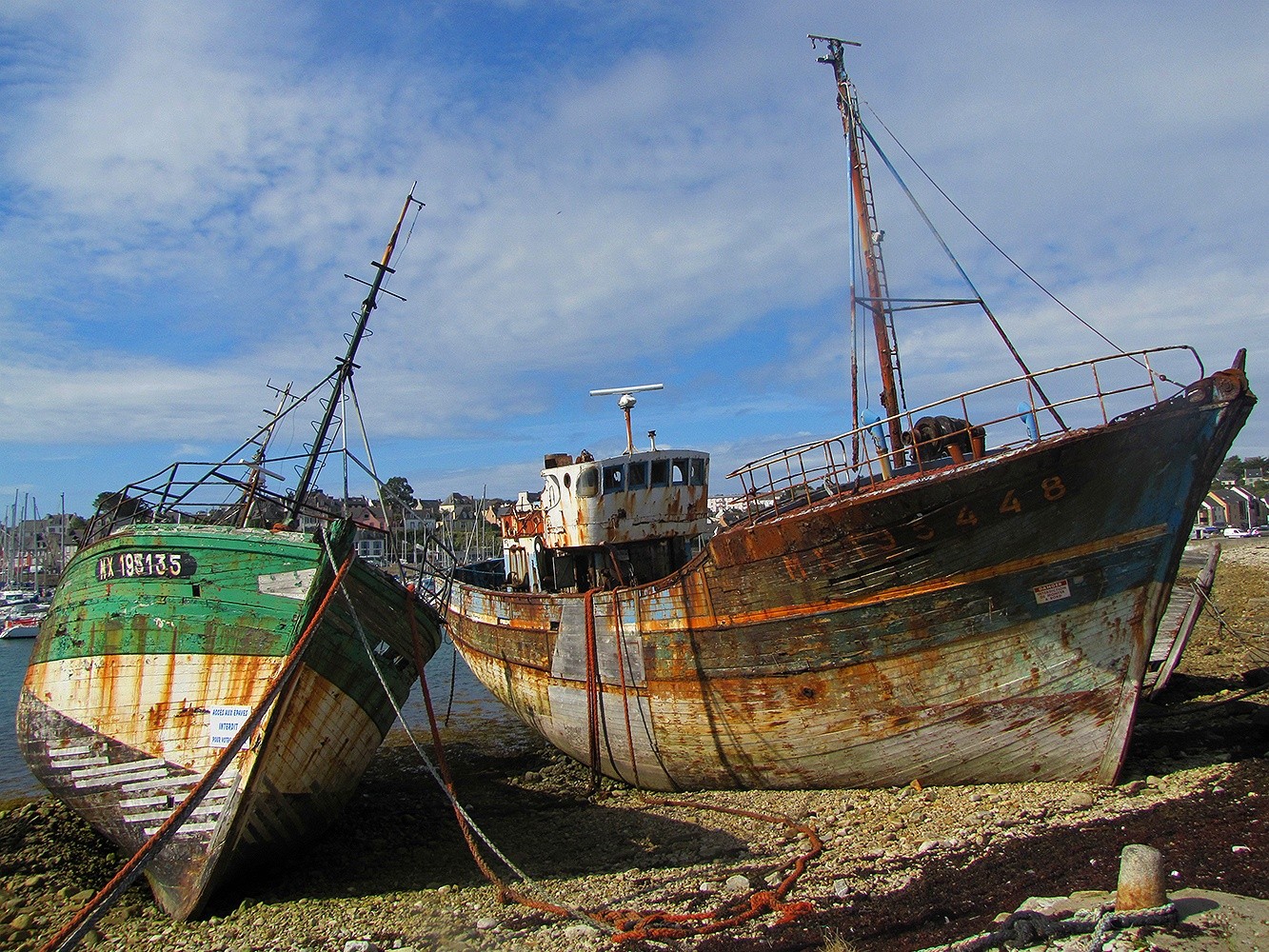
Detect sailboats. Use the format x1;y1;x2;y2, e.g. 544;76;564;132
0;487;69;640
391;511;458;616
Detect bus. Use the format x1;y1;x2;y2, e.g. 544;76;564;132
359;555;389;568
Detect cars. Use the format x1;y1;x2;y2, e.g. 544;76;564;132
1189;521;1269;540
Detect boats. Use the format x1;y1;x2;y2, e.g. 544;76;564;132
14;177;461;927
402;25;1264;802
